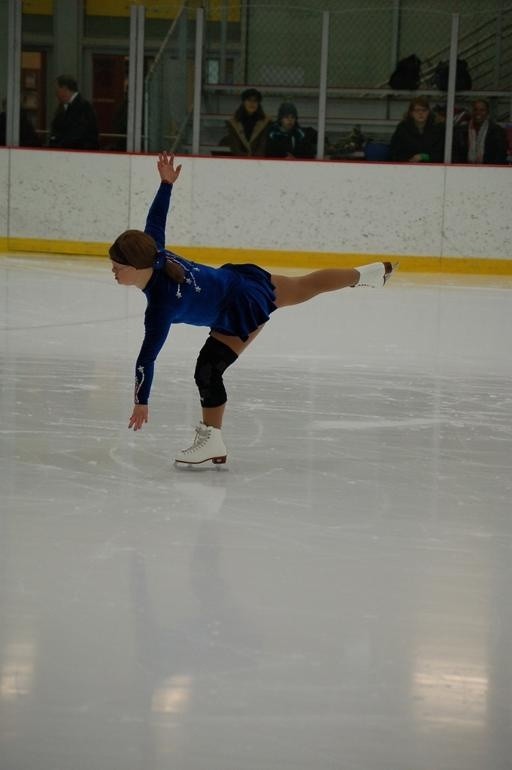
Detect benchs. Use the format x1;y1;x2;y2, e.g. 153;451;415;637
178;102;511;161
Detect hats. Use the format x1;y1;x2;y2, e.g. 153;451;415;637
241;89;262;101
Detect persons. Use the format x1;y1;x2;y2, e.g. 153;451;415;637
108;149;398;473
451;98;506;164
224;88;272;157
49;72;97;150
388;96;445;164
265;101;316;159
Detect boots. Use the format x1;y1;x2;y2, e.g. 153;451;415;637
174;422;227;464
354;261;392;289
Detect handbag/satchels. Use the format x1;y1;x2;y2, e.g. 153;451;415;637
390;55;421;89
438;59;472;90
